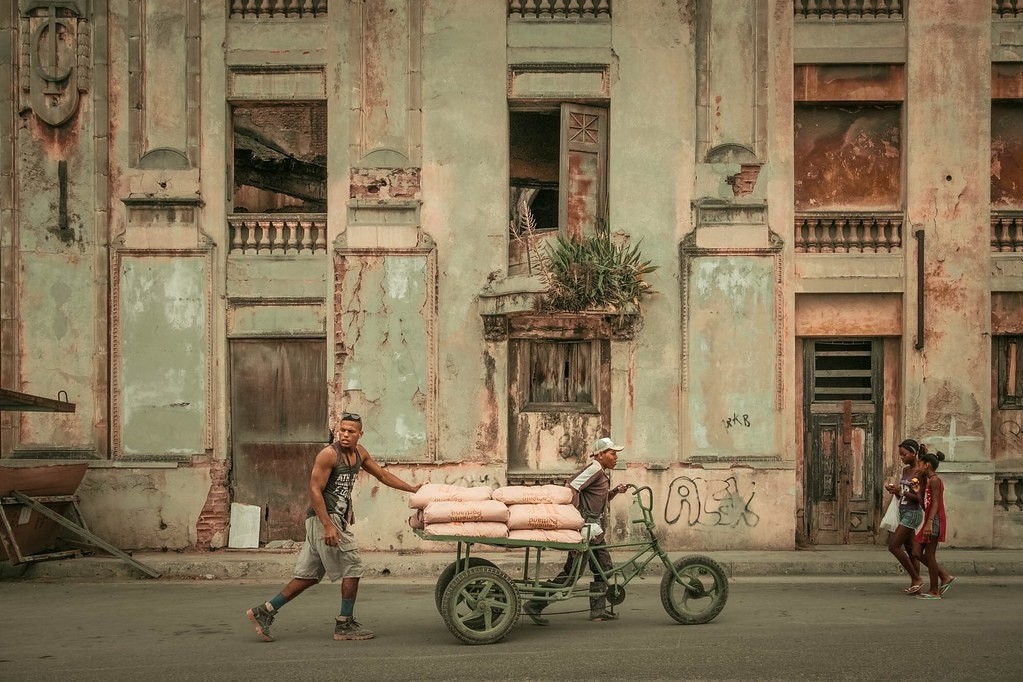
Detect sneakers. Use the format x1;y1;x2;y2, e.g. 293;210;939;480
334;615;373;640
247;601;278;642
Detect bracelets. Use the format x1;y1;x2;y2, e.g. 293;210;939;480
927;516;935;522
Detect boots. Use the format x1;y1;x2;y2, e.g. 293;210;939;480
590;582;616;621
523;579;564;626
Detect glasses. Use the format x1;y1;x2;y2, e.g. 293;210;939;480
343;413;359;419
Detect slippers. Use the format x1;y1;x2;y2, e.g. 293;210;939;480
939;576;956;596
899;584;924;594
916;593;941;600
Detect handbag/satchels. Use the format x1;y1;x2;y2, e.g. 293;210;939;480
879;494;900;533
578;505;603;543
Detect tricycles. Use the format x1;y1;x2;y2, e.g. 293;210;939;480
412;484;729;645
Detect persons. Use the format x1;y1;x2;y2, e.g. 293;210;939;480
246;413;429;642
522;437;629;626
884;439;956;600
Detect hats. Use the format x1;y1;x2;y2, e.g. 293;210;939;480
590;438;624;457
